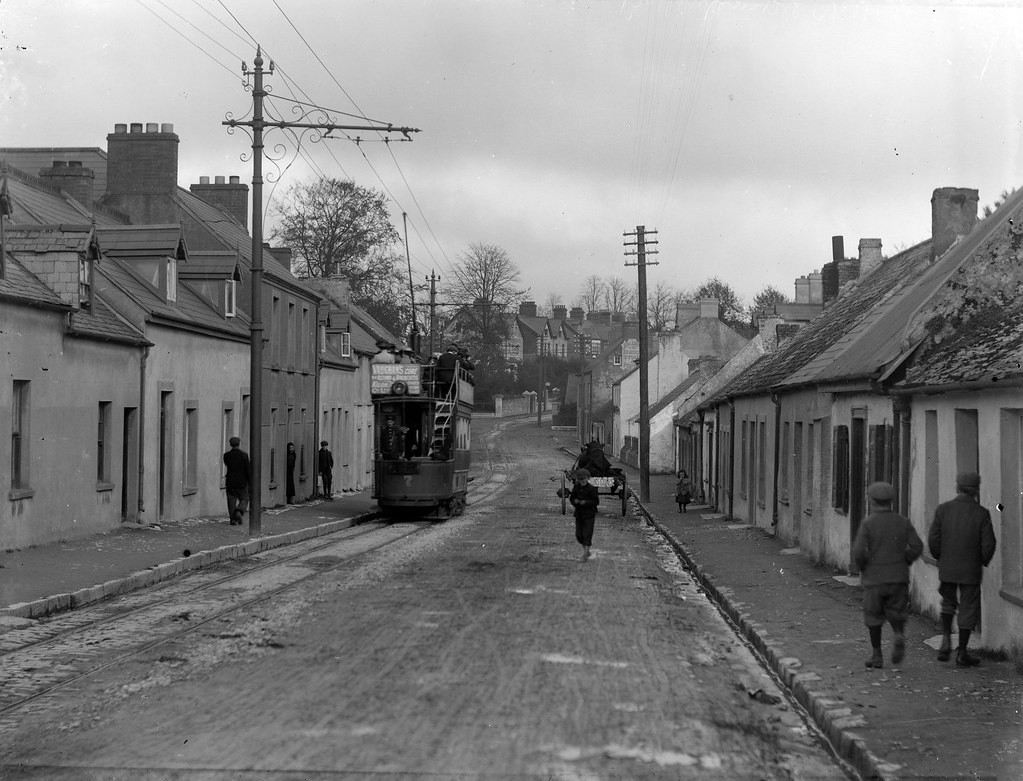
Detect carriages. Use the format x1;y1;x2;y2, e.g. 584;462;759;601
556;441;633;517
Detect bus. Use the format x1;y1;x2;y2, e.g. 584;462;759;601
369;358;476;523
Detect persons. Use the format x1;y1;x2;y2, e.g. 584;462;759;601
570;469;599;562
676;470;692;513
577;445;588;469
852;482;924;667
928;472;996;667
319;441;333;500
286;442;296;505
379;417;414;460
224;437;250;525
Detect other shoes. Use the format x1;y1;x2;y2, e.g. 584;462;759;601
891;634;905;665
287;500;295;505
957;655;981;667
323;494;333;501
580;555;587;563
678;508;682;513
936;638;952;662
865;654;883;669
230;519;236;525
234;511;243;525
583;552;591;557
683;508;686;513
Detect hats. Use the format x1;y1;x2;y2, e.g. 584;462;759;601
866;482;896;502
576;468;590;480
229;437;240;446
956;471;980;487
321;441;329;447
